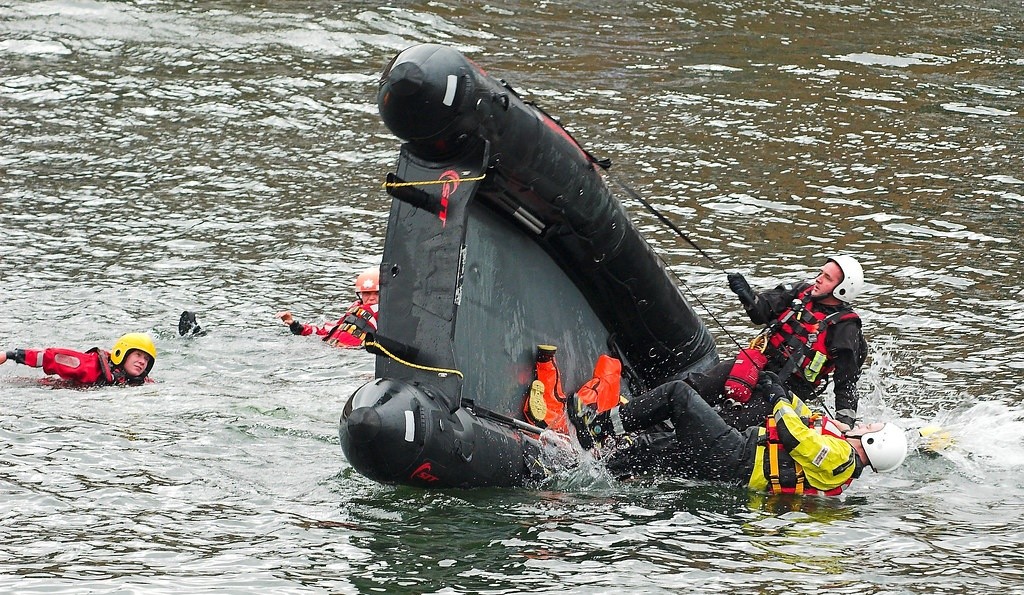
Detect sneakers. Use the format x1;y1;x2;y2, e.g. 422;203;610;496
568;392;597;452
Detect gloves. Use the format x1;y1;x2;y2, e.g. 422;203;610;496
728;273;756;304
837;415;856;434
756;371;788;406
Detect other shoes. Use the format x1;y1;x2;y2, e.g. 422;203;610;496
528;380;546;421
536;344;557;363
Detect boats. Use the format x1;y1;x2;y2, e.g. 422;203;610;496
339;42;720;492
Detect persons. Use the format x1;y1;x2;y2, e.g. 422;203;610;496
274;269;380;348
682;255;868;431
565;370;908;497
0;332;156;382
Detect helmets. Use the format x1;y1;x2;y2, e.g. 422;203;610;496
861;422;907;473
824;256;864;304
355;267;380;293
919;426;955;457
110;333;158;382
